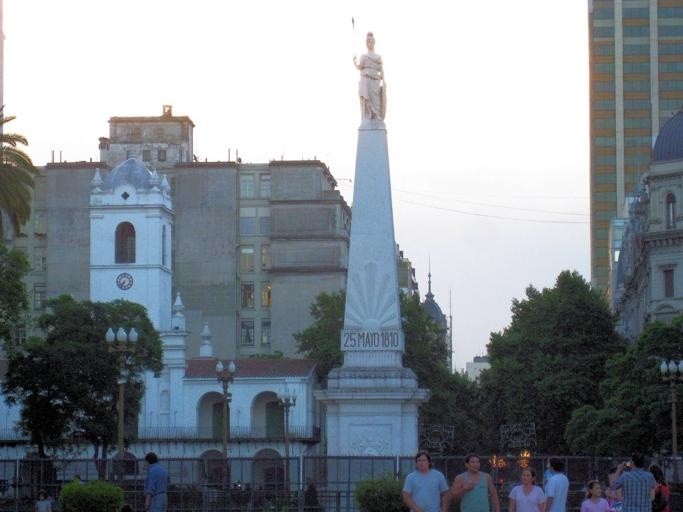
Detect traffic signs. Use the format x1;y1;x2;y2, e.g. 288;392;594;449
275;386;296;493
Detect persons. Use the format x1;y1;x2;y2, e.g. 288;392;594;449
648;462;670;511
604;466;623;512
579;479;618;511
351;32;385;122
401;450;451;512
34;488;52;511
225;475;321;512
444;452;501;512
609;452;657;511
542;457;570;512
144;451;167;511
506;466;546;512
72;473;86;486
540;454;553;492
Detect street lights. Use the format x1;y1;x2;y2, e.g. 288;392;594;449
104;327;138;487
214;360;238;492
661;358;682;483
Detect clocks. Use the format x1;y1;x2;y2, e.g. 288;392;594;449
115;273;133;290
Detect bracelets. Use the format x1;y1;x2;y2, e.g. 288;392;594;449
616;468;621;472
